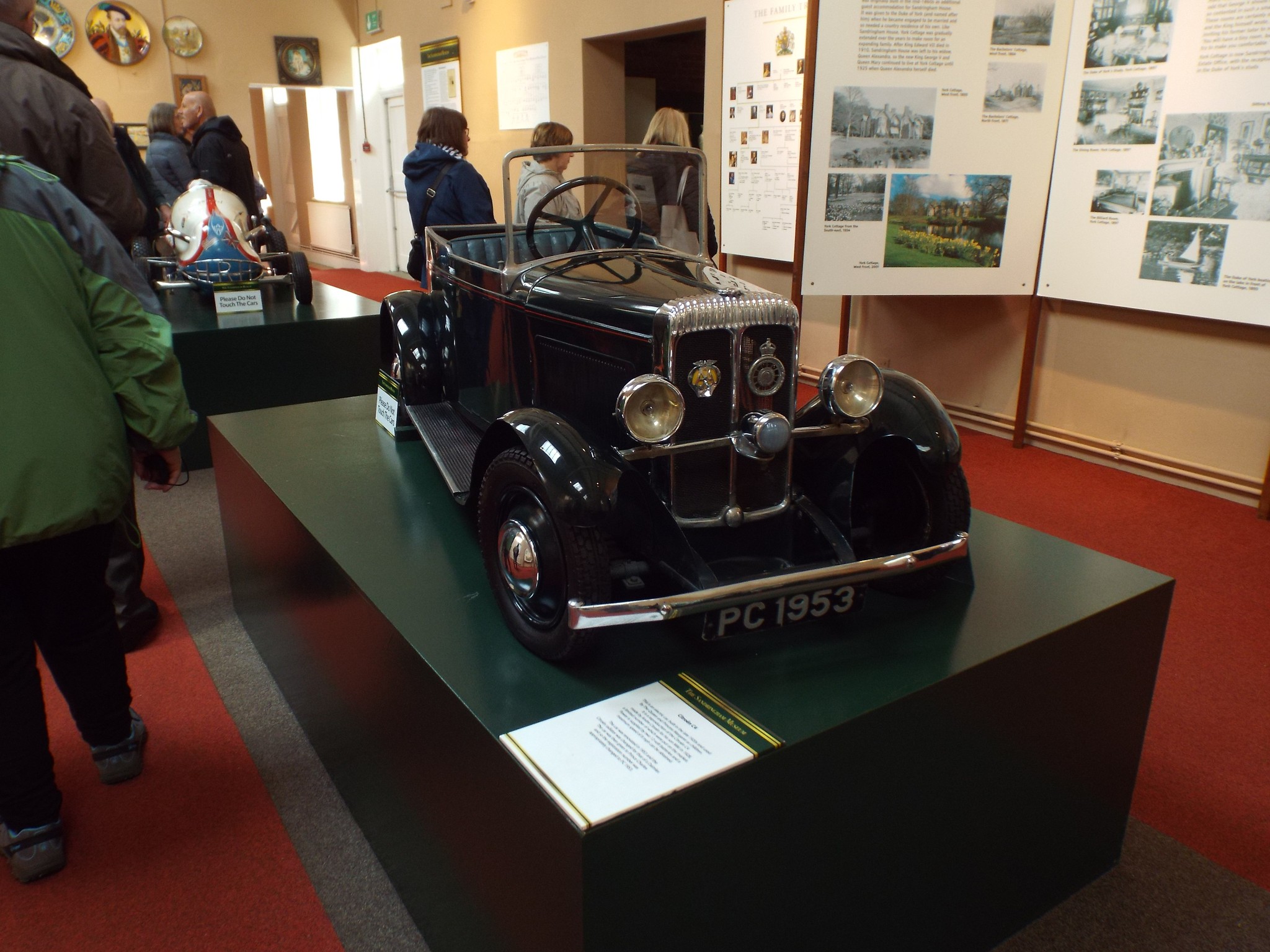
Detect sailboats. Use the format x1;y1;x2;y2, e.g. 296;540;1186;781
1156;226;1202;271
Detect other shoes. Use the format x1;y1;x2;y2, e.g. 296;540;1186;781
120;595;158;651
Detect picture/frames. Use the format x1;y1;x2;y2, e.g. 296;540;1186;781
274;36;322;86
174;74;209;107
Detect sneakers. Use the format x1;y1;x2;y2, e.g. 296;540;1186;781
0;813;66;882
91;707;145;781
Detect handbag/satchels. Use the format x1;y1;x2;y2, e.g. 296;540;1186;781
659;165;701;257
407;240;425;282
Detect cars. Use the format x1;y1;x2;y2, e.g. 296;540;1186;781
130;178;313;306
379;144;977;666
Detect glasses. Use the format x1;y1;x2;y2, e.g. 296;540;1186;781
463;127;469;136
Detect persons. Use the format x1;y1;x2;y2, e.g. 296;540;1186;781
751;106;757;119
730;155;737;167
146;91;267;222
402;106;498;290
798;59;804;74
751;152;757;164
514;122;582;225
0;2;195;882
764;64;770;77
767;106;772;118
729;172;735;184
730;108;735;118
781;111;785;121
625;108;718;269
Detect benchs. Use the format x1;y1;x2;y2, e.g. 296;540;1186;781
450;224;638;269
